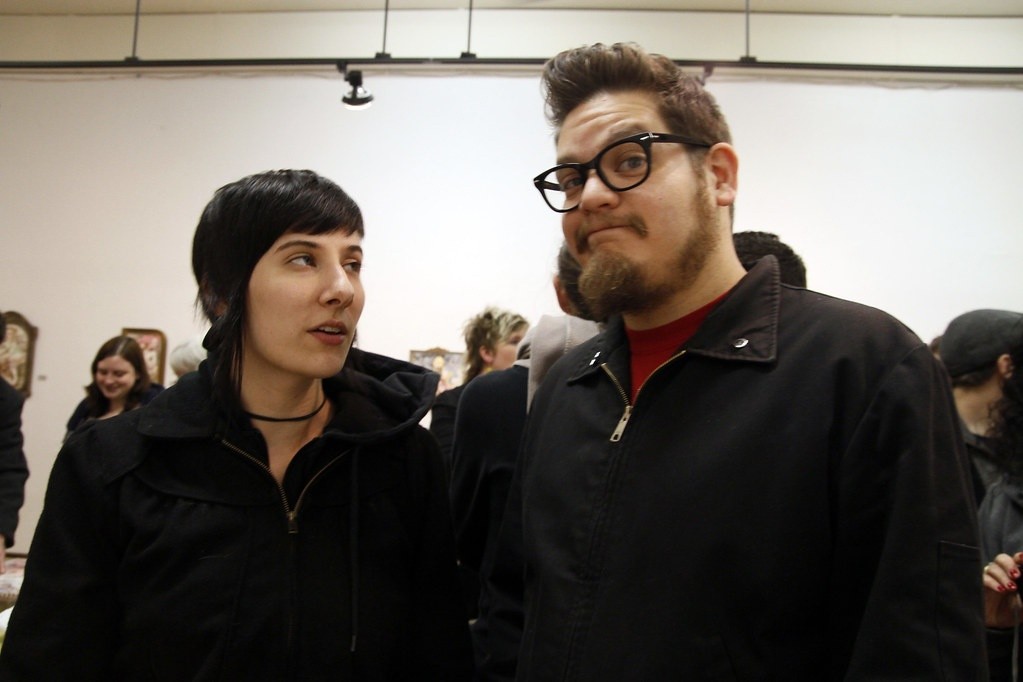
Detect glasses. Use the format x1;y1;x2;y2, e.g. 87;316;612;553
533;132;710;213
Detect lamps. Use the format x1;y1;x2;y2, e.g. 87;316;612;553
340;69;374;113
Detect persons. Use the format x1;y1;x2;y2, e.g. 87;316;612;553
432;307;530;409
731;230;806;288
64;336;165;440
0;168;477;682
451;239;605;605
0;315;30;573
973;309;1023;682
936;309;1023;489
170;339;207;380
475;42;989;682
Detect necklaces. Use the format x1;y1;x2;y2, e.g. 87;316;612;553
247;392;326;422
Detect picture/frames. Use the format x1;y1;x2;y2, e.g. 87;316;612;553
409;347;467;391
122;326;166;387
0;310;38;399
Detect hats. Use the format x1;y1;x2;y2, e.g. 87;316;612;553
938;309;1023;376
170;340;208;373
